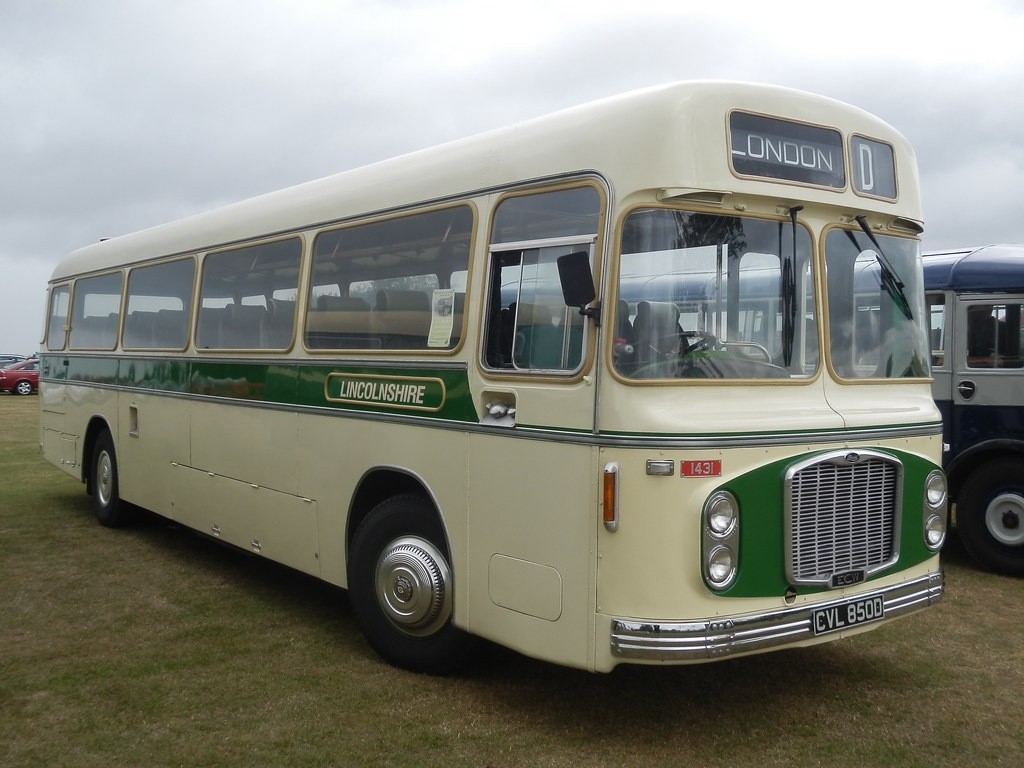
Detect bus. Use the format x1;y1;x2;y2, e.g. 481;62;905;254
500;245;1024;577
39;81;948;672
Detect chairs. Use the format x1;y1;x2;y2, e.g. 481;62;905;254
376;289;430;350
510;300;694;379
261;298;296;350
83;316;108;348
219;304;265;349
153;309;185;347
187;307;225;349
317;295;371;312
50;315;70;349
129;311;157;348
110;312;131;348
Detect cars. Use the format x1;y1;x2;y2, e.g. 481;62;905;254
0;360;41;395
0;354;40;370
